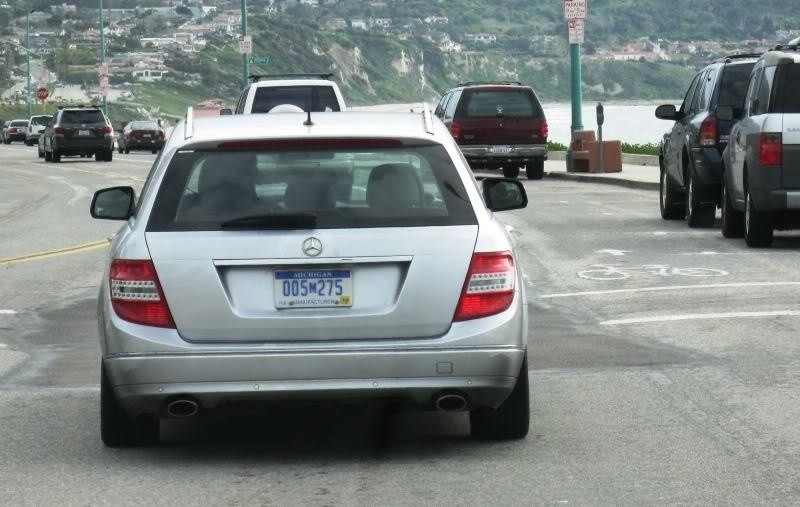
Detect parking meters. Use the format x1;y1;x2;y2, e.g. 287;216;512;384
595;103;606;171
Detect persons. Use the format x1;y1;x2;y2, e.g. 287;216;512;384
281;172;337;208
208;152;259;201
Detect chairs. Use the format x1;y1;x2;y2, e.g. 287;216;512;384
197;161;261;208
361;161;425;213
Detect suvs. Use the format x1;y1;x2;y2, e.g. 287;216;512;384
218;72;349;184
717;37;800;247
659;51;762;229
24;115;57;146
43;104;114;162
433;81;551;181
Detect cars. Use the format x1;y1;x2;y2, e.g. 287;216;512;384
116;121;164;154
2;120;12;136
86;106;534;450
4;119;31;144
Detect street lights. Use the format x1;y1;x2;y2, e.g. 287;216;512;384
0;4;32;118
